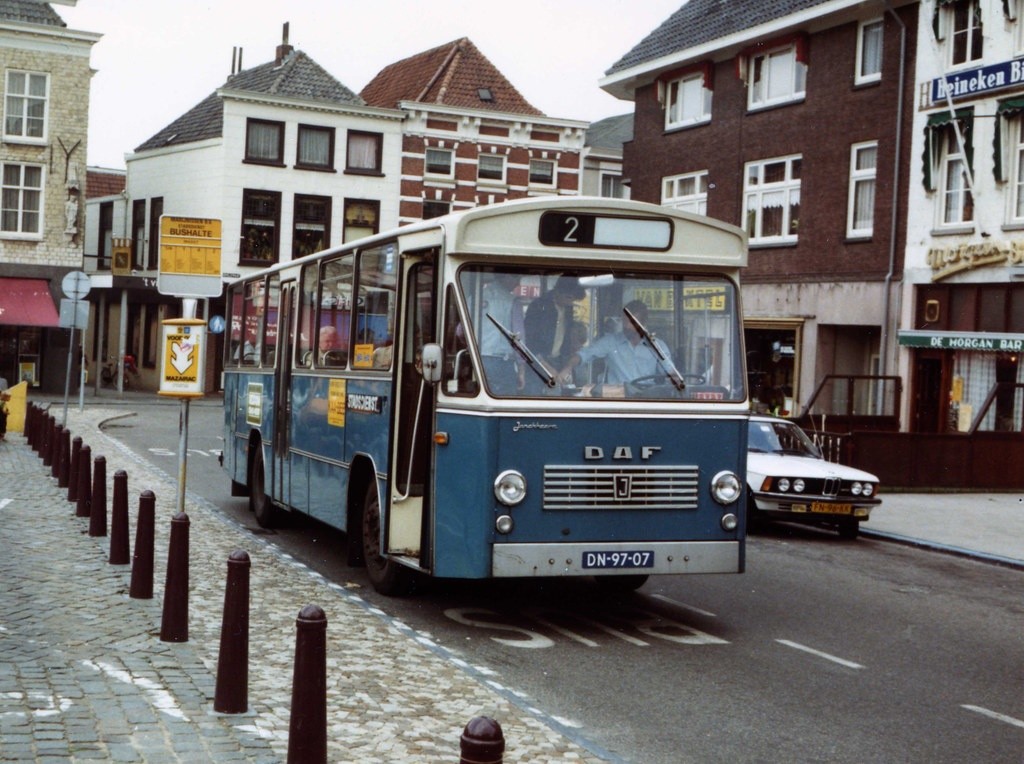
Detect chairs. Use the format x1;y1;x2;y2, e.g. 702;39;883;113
225;347;345;366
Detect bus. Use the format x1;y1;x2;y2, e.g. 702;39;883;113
219;195;747;599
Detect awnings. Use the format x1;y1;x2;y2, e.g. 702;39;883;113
0;277;60;328
899;328;1024;353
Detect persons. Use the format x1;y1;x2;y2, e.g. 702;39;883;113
761;369;793;397
77;343;88;387
358;328;374;343
550;321;605;385
233;327;255;361
65;195;78;228
265;349;275;364
306;326;340;366
558;298;686;395
371;323;420;368
466;274;527;395
523;273;586;397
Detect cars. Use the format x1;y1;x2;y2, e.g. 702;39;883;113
745;414;882;541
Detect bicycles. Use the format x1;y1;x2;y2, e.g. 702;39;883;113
101;355;131;391
